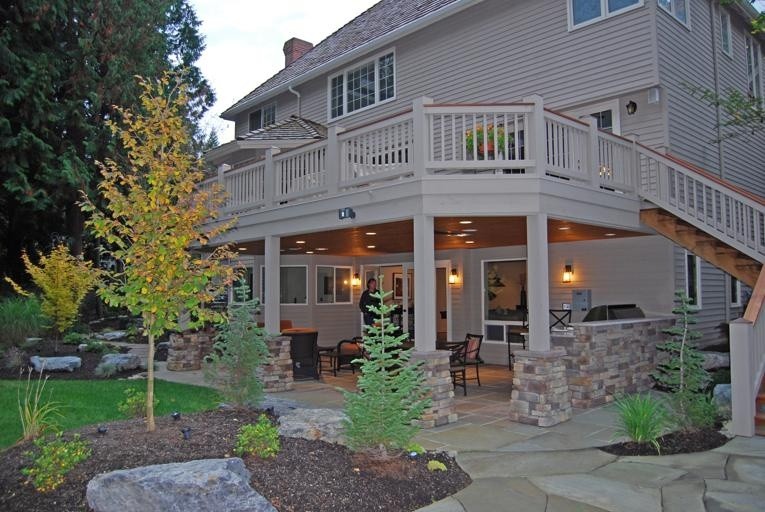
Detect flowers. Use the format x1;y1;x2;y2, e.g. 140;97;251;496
465;125;513;152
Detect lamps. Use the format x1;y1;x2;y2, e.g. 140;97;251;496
338;206;356;219
351;272;361;288
449;266;457;286
562;262;571;283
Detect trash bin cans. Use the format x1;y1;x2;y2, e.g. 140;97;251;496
284;327;320;381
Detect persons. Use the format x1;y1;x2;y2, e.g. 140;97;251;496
359;278;380;330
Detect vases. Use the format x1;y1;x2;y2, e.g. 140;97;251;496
479;141;495;153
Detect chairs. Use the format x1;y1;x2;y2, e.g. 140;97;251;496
284;325;483;395
506;308;571;367
